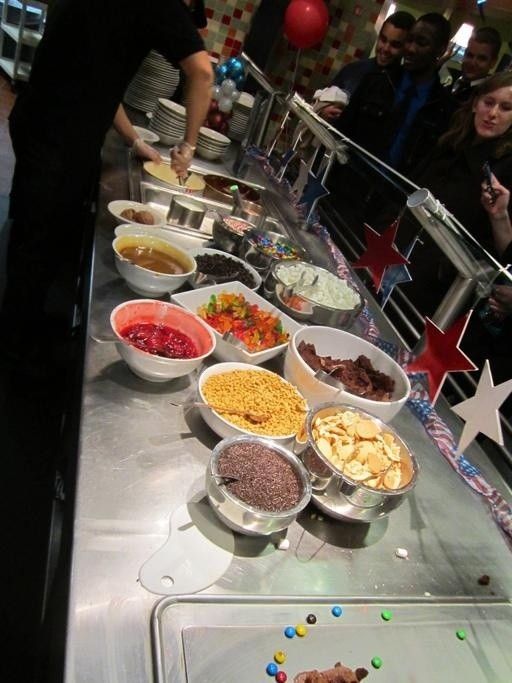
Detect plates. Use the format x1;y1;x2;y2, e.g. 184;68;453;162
123;50;181;112
229;90;256;134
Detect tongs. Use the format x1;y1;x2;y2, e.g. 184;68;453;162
314;364;370;398
280;271;319;296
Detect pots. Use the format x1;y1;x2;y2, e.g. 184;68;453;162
203;174;261;205
264;257;366;330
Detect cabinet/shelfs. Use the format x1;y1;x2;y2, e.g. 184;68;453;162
0;0;48;91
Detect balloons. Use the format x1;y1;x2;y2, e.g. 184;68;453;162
203;56;245;136
284;0;330;48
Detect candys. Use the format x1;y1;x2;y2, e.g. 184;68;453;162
254;233;302;261
197;290;290;351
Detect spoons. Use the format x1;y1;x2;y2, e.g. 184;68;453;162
193;400;271;422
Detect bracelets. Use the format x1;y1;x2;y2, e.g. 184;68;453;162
132;138;143;151
182;141;196;151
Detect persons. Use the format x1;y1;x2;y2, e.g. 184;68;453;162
2;0;216;281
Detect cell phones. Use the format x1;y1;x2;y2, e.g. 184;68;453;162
482;160;496;201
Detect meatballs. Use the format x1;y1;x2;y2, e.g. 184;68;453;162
119;208;155;225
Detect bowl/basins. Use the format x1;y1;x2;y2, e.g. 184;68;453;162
107;193;309;385
196;363;311;445
284;326;412;422
295;401;421;525
206;436;314;538
147;98;233;161
131;124;160;145
